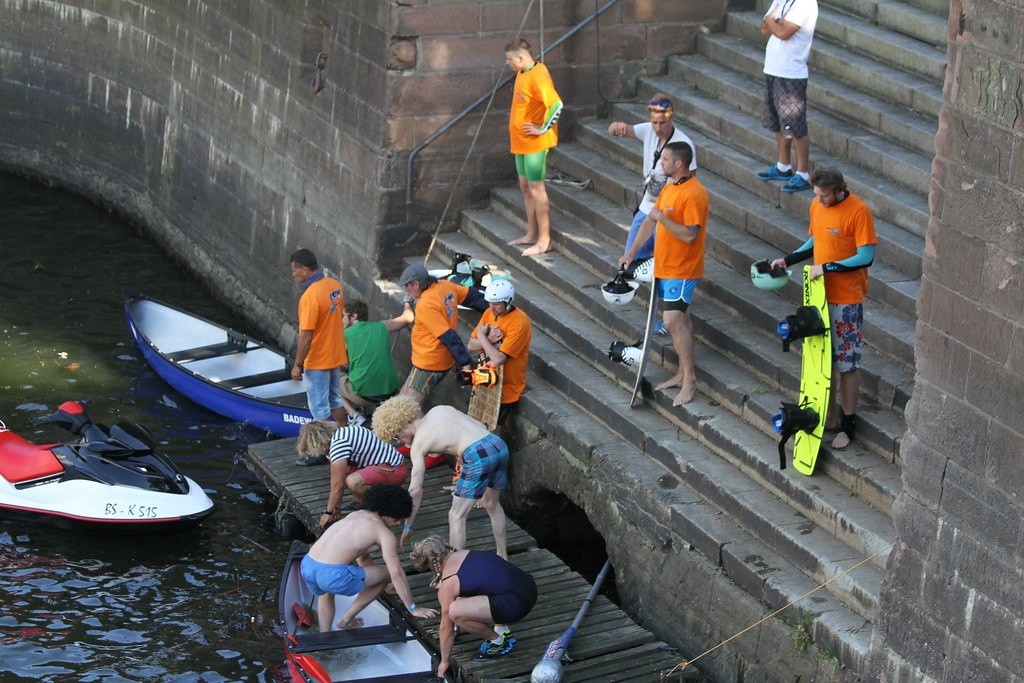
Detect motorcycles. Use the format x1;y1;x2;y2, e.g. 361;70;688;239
0;400;218;523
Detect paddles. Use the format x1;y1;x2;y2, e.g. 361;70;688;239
286;603;313;645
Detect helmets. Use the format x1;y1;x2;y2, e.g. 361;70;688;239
485;279;515;321
750;259;789;291
601;281;640;306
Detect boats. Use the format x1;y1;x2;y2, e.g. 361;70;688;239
280;540;457;683
124;291;335;437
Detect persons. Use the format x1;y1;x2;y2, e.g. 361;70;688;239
337;292;415;426
756;0;818;192
769;167;878;450
608;94;698;338
297;418;408;527
618;141;708;407
299;482;441;634
442;278;532;508
289;248;346;467
371;395;511;590
385;264;488;470
411;535;537;678
504;38;563;256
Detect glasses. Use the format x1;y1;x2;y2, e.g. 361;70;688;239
650;102;673;113
340;312;351;318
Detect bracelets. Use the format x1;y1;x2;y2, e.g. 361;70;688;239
323;510;333;515
407;601;416;612
402;525;413;531
402;300;412;307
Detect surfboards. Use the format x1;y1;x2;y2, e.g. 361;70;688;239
770;266;833;475
607;221;657;407
425;252;510;308
451;337;505;491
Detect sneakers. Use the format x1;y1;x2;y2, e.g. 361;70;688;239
777;305;824;352
769;402;819;469
624;254;656;283
756;164;793;180
455;368;498;389
436;253;472;282
502;629;516;644
467;265;493;294
781;175;811;191
608;341;642;366
472;635;513;662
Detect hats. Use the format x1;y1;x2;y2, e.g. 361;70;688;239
396;262;428;286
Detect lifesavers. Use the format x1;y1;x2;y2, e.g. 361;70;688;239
263;513;293;537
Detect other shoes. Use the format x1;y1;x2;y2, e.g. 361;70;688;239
398;445;411;458
652;323;671;337
347;411;366;426
295;455;329;465
424;454;446;469
